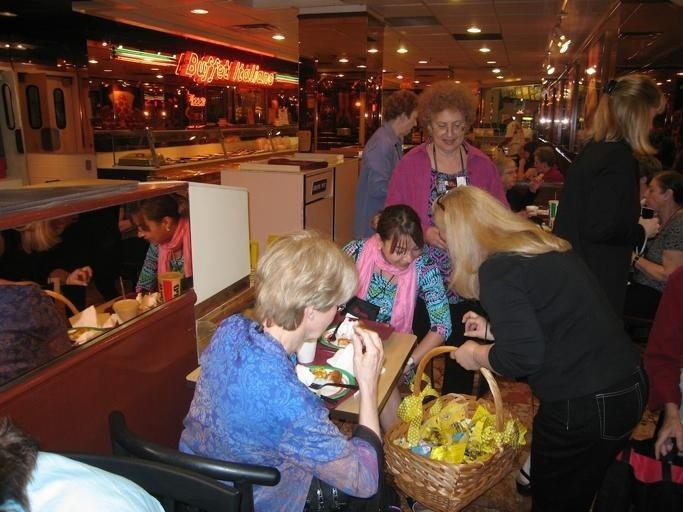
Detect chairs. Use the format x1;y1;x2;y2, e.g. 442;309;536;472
104;409;283;512
45;449;243;512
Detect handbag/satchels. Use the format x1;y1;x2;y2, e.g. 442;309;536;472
591;438;682;512
343;425;400;512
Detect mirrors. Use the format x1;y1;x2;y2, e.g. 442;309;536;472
367;11;384;141
0;188;194;397
411;67;447;145
447;67;453;83
297;12;367;158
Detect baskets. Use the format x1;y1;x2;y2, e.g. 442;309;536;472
381;346;519;512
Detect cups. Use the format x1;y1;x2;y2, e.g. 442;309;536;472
297;339;317;364
547;199;558;222
157;272;181;304
113;298;138;321
640;206;655;219
525;205;539;212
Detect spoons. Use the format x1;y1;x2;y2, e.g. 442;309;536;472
307;380;359;392
329;320;343;341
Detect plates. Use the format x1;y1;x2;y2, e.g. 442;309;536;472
66;327;105;342
318;324;374;351
301;365;355;400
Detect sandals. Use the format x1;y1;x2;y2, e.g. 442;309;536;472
516;468;532;495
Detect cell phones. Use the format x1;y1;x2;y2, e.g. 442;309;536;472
642;208;655;219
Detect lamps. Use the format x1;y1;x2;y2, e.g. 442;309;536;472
539;24;570;86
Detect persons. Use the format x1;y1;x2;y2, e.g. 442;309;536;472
126;192;190;290
0;413;166;512
0;285;74;384
0;215;93;316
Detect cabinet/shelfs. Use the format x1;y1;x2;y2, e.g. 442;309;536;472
93;121;296;183
